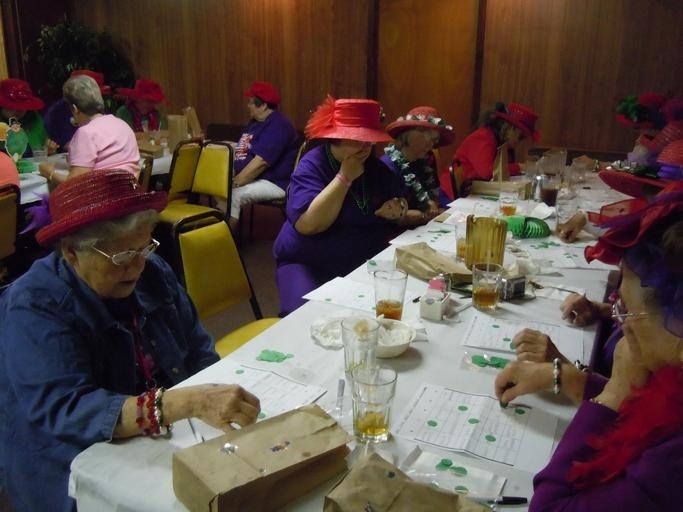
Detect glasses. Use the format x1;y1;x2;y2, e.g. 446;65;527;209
82;237;160;265
613;300;672;322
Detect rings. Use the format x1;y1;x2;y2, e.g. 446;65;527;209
560;231;566;234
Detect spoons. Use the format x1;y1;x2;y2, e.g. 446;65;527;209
527;280;577;295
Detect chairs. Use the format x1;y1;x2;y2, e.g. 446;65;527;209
1;184;21;281
240;139;306;234
172;211;285;359
138;159;153;191
159;141;234;242
450;160;464;198
164;139;203;194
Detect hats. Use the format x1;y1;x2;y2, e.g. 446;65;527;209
119;80;166;103
36;170;168;245
245;82;281;102
71;69;110;95
616;92;663;130
305;98;392;142
0;79;44;110
490;103;541;141
637;121;683;152
386;107;455;145
601;140;683;196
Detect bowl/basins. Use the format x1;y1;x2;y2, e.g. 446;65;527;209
352;319;416;358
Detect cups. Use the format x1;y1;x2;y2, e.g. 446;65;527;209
466;214;507;273
543;155;561;178
526;161;536;179
342;318;380;385
499;191;518;216
473;261;504;309
575;163;586;183
350;361;398;445
552;147;567;189
456;225;468;261
540;176;557;208
374;269;409;324
34;149;47;162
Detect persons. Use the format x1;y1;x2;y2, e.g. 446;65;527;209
558;96;683;241
39;77;142;180
510;184;682;405
0;152;21;284
44;68;109;154
441;99;543;201
1;79;42;158
113;78;165;132
36;170;258;509
494;211;683;508
213;81;306;230
385;107;452;222
276;95;397;315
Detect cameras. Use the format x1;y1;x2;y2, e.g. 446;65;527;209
500;275;526;300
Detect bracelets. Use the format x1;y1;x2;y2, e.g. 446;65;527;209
335;175;349;187
578;209;591;224
553;358;562;395
399;199;406;221
136;386;174;437
592;157;599;170
574;360;581;370
49;168;54;179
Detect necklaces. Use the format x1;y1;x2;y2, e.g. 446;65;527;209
322;140;372;215
